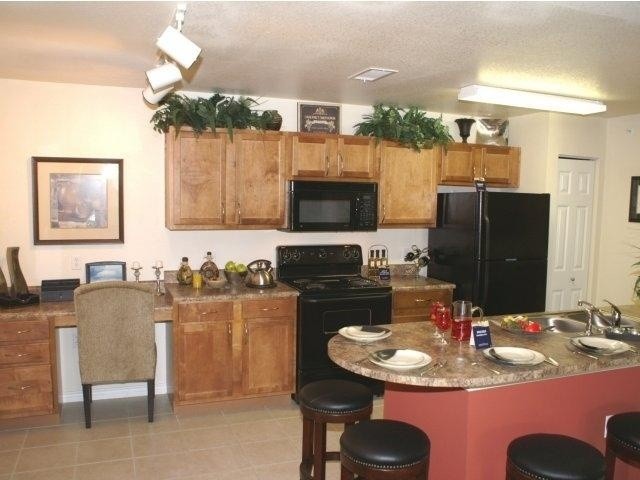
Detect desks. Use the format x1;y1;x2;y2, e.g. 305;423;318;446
328;304;640;480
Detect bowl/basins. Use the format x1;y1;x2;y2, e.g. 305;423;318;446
224;271;248;284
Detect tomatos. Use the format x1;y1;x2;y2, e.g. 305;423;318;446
522;320;542;333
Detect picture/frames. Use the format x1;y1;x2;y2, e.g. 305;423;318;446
31;156;123;245
628;176;640;222
298;102;342;133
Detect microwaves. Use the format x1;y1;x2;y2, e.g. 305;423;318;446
277;176;379;233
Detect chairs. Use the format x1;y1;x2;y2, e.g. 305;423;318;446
74;281;157;429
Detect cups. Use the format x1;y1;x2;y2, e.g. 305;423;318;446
452;301;483;343
193;271;202;289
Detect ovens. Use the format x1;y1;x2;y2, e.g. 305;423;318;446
298;293;391;395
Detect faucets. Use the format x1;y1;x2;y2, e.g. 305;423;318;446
579;300;603;333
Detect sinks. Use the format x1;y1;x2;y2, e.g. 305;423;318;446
525;315;600;334
568;313;640;329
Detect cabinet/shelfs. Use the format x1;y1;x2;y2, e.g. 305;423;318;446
165;125;291;231
285;131;380;181
437;142;521;188
377;138;438;228
392;285;456;323
165;284;299;407
0;314;63;431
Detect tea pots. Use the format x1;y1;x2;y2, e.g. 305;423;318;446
247;260;273;285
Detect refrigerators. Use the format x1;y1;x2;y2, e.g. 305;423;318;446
428;183;552;315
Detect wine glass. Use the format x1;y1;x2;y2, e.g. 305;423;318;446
436;307;451;347
429;300;444;338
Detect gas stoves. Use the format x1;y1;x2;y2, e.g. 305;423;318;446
277;245;392;293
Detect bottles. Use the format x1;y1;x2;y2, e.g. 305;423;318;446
370;249;387;272
200;253;218;284
176;258;194;285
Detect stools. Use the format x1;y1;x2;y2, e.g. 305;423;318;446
339;419;430;480
605;411;640;480
506;433;607;480
299;379;372;480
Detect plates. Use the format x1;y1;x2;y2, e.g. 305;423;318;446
582;338;623;350
348;326;385;337
340;326;392;342
489;348;535;362
491;319;548;334
484;347;545;366
369;351;432;369
572;338;629;355
378;350;424;364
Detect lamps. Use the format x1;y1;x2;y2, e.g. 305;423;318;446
143;84;174;105
155;11;202;70
458;85;606;116
145;54;182;93
